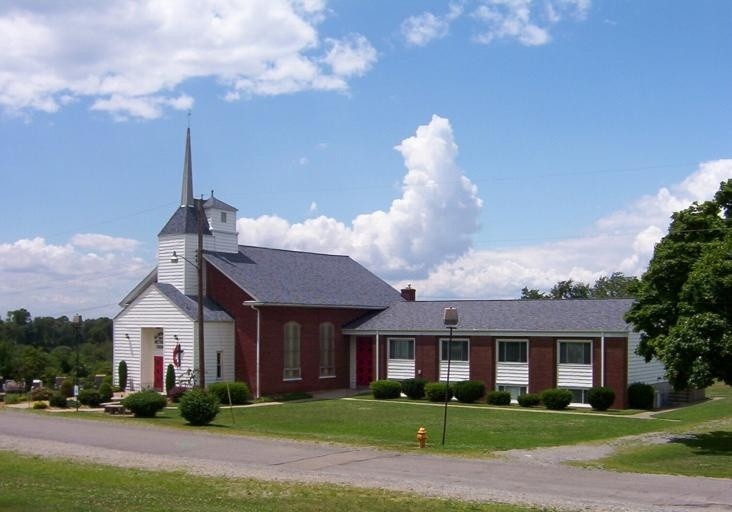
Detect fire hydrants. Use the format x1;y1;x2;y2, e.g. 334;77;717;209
416;426;429;448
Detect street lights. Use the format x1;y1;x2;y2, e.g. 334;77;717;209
441;307;458;446
170;250;204;387
